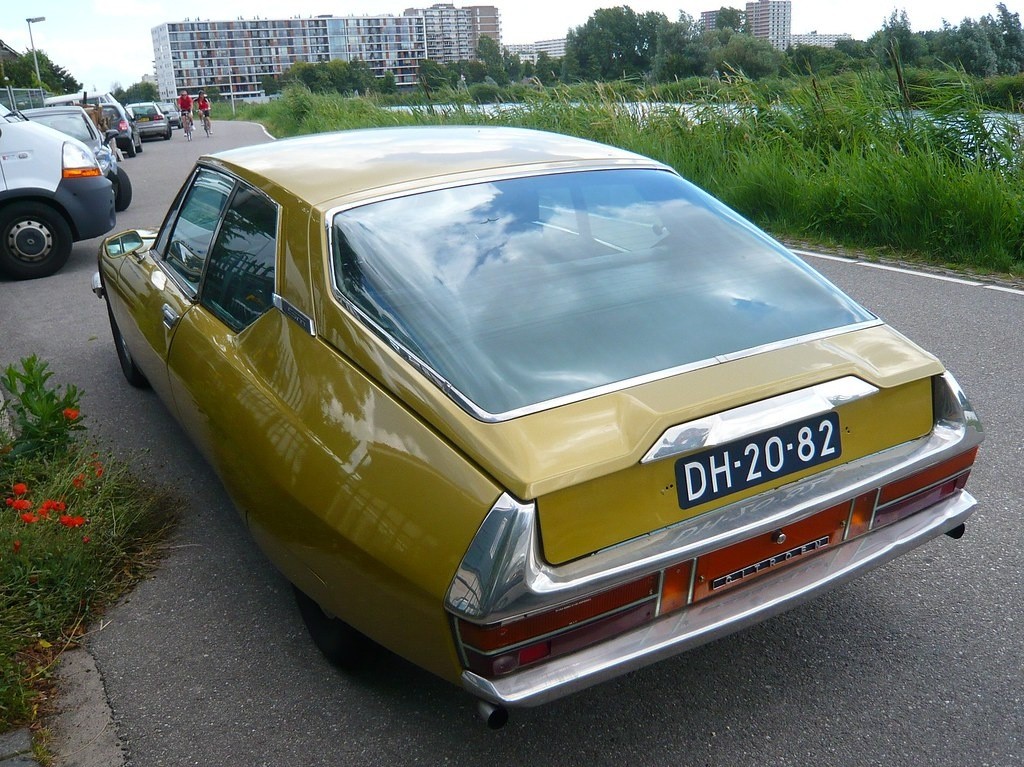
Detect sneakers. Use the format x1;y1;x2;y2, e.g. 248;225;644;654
190;126;195;130
183;133;188;137
210;131;213;134
201;120;204;125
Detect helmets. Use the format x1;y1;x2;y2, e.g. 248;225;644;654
180;90;187;95
198;90;204;94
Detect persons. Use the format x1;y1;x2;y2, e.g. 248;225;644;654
177;90;195;137
193;90;213;134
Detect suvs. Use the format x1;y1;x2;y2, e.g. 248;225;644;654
124;100;172;141
101;103;143;158
0;105;116;280
18;106;133;213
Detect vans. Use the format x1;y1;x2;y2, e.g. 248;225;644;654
44;91;119;106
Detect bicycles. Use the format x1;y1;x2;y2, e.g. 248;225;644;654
196;108;210;137
181;109;193;141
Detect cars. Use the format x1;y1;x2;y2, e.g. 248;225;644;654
157;103;183;129
89;126;984;726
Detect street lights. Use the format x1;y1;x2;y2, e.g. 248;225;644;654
26;17;45;104
